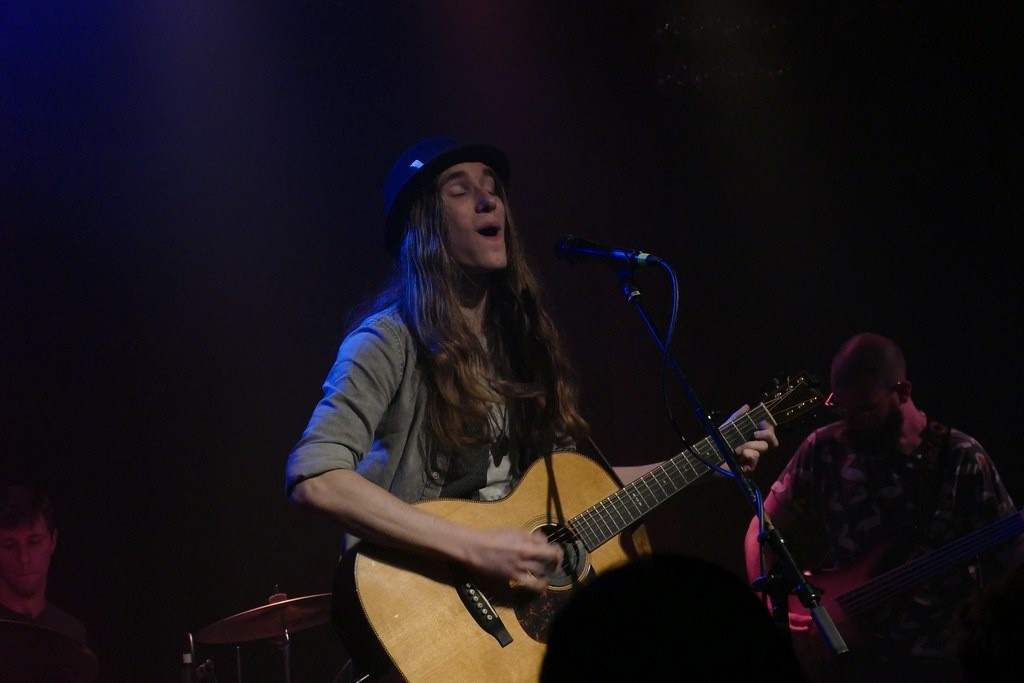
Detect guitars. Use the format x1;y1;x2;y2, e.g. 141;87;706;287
773;509;1024;683
341;372;825;683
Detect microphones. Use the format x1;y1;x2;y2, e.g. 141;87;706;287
183;637;192;683
554;234;663;266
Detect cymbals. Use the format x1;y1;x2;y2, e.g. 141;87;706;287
192;589;339;643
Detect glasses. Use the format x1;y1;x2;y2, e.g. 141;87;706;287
824;382;903;419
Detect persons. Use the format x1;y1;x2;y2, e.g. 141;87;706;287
0;485;100;683
745;333;1023;683
285;137;779;683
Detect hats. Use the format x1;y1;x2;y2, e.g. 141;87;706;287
381;131;509;224
